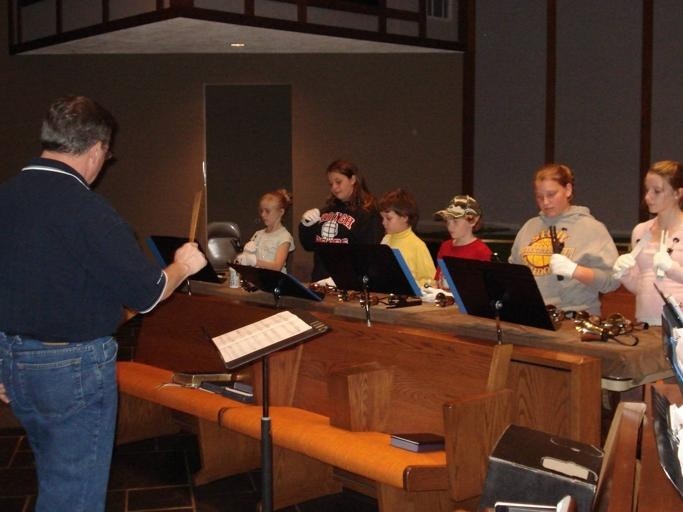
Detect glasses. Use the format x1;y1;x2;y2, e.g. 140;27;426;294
102;147;113;160
535;186;564;200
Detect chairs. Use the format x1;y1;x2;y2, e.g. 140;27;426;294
0;382;206;512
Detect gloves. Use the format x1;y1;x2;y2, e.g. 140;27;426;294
244;241;257;253
612;253;636;280
237;253;257;268
549;253;577;280
300;208;321;227
652;251;673;277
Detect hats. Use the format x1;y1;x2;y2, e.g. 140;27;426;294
432;193;482;220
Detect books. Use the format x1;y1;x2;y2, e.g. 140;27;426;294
174;371;252;405
211;310;313;363
388;432;445;451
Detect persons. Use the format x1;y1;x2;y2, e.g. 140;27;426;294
363;188;437;297
431;192;494;293
612;158;683;325
505;165;621;315
0;93;209;512
297;155;379;291
234;187;295;278
